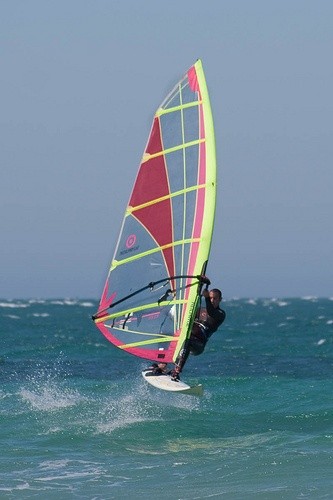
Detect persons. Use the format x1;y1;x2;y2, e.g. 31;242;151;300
152;288;226;376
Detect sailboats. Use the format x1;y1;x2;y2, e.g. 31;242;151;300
90;55;219;391
